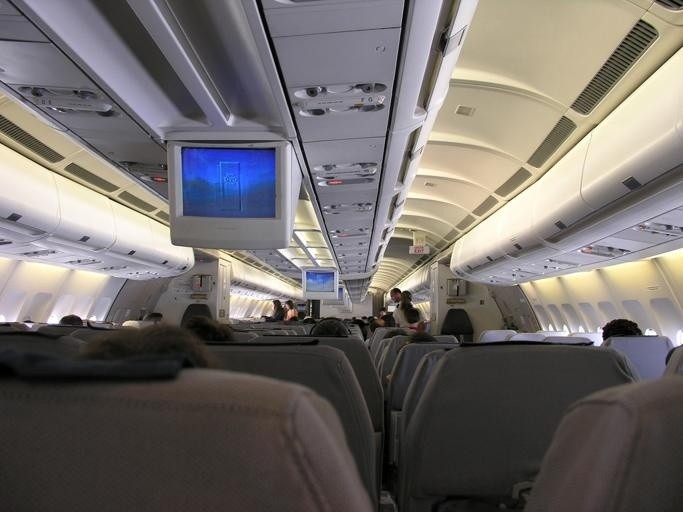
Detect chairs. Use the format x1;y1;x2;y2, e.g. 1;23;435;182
0;312;683;511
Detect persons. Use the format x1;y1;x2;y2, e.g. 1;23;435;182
285;301;299;321
601;318;644;342
351;288;431;348
59;312;246;370
271;301;285;321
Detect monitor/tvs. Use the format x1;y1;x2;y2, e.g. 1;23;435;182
302;267;339;301
322;284;346;306
166;139;303;251
